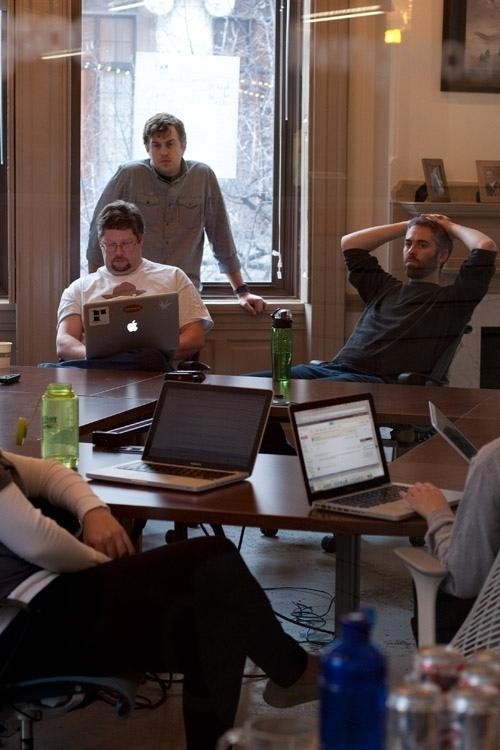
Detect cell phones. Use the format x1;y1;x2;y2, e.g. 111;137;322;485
0;374;20;383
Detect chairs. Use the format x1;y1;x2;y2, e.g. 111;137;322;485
1;644;132;749
56;274;210;374
309;325;473;387
389;545;499;664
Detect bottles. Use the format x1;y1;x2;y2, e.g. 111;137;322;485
270;308;292;381
318;603;390;750
40;382;80;471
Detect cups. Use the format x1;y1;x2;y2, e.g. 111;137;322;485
218;713;314;749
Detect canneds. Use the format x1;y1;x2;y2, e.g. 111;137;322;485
386;647;500;750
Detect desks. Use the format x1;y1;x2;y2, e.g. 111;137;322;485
2;362;498;656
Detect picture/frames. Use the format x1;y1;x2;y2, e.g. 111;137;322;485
473;158;500;204
413;154;452;204
438;1;500;94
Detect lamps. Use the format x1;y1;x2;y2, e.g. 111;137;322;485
42;47;89;62
300;1;394;30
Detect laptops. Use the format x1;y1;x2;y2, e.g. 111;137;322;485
83;293;180;359
86;381;274;492
428;400;478;463
288;393;464;521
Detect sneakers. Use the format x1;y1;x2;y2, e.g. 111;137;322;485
263;655;320;708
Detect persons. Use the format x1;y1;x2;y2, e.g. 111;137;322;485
84;112;267;318
0;445;325;750
55;199;215;552
238;214;497;386
398;437;500;656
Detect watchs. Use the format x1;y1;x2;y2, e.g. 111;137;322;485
233;282;249;296
